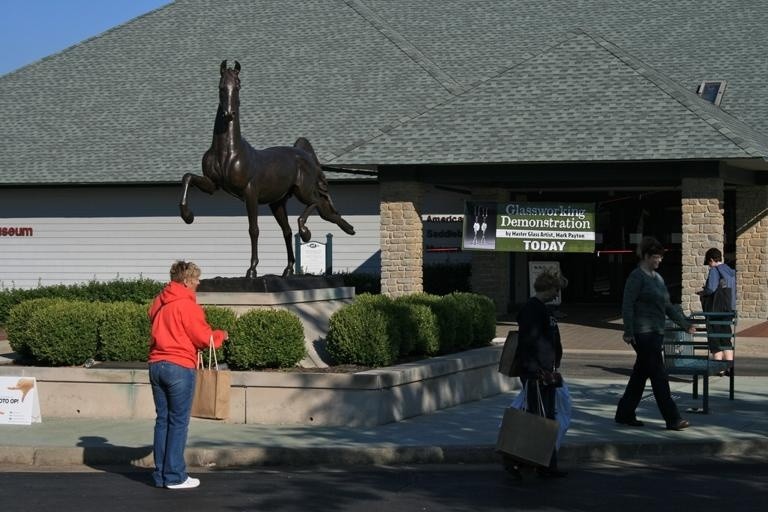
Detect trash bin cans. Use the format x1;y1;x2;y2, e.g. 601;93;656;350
663;318;694;369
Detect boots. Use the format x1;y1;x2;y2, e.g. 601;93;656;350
666;420;689;429
615;416;644;426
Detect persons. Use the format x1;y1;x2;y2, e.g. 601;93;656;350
501;272;568;480
695;247;736;376
615;237;697;430
147;260;229;489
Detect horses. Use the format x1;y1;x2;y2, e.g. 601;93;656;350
179;60;356;277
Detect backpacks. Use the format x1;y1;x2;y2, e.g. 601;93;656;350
498;330;522;377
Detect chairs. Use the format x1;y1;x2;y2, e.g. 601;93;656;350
662;310;737;415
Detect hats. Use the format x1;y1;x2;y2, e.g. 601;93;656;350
704;248;721;265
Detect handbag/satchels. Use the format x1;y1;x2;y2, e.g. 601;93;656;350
495;406;560;467
191;369;232;420
700;287;732;321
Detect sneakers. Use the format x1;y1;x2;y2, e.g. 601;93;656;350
166;476;200;489
